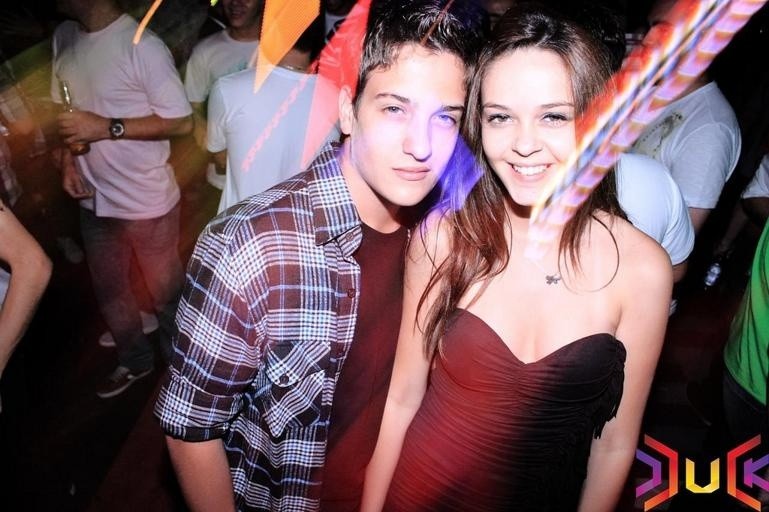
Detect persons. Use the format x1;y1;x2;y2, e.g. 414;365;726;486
575;10;695;284
720;148;769;512
152;5;480;511
1;196;52;382
360;11;672;512
633;0;743;235
50;1;195;398
183;0;348;215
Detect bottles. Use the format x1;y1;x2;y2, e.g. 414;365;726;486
703;261;721;288
56;80;90;156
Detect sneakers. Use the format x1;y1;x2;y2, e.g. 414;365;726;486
98;311;160;348
97;363;154;398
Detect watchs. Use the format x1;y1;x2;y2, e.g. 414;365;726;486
109;117;124;140
